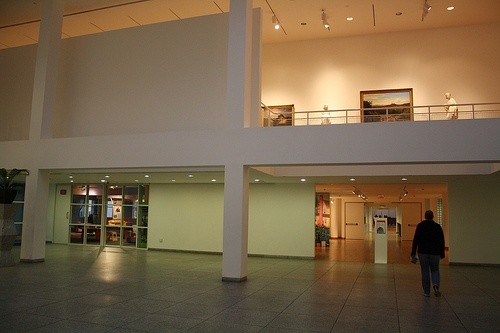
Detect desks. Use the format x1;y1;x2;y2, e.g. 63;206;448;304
78;226;134;243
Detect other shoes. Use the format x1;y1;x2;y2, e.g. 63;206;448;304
433;285;440;297
423;292;431;297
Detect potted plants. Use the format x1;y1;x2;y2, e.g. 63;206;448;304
0;167;29;204
315;226;330;247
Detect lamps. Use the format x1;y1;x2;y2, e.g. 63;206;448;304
271;15;280;31
320;11;331;29
423;2;432;12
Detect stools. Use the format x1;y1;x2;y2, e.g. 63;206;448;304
106;231;118;242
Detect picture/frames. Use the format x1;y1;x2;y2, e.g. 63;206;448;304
261;105;295;127
360;88;414;123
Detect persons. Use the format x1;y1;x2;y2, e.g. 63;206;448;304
320;105;331;123
444;92;458;119
411;210;445;297
